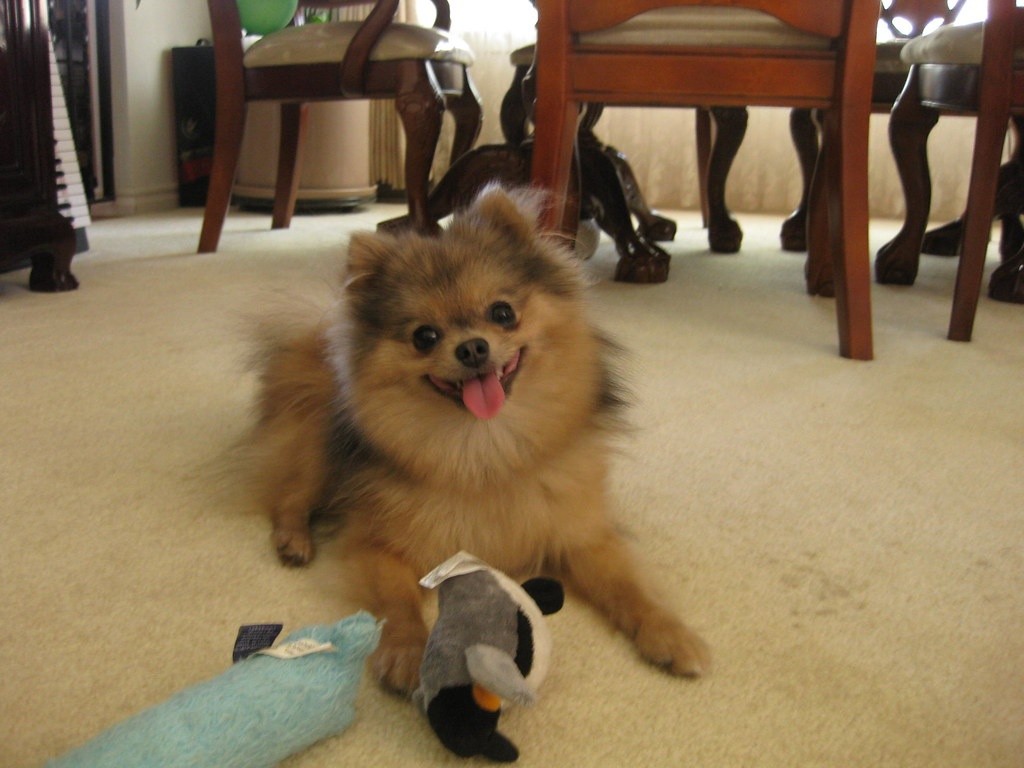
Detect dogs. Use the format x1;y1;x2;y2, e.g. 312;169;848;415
180;173;717;701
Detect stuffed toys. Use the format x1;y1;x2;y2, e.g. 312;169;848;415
45;608;388;768
409;552;573;763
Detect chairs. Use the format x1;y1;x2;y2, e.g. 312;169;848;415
501;0;1024;363
199;0;483;253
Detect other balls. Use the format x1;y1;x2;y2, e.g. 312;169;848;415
234;1;299;38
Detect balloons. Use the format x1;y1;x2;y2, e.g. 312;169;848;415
237;0;298;39
